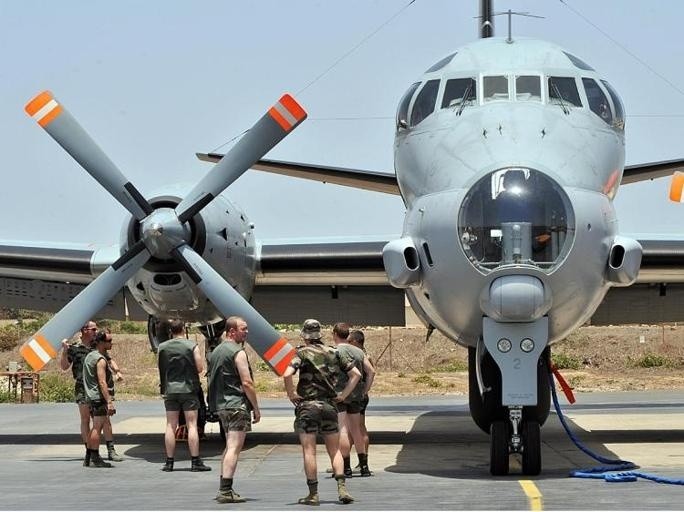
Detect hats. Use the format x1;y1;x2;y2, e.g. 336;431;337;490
299;318;324;340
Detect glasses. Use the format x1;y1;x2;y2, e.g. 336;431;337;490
88;328;98;331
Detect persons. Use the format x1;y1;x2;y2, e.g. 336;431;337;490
284;318;361;506
59;320;126;468
211;315;260;505
326;323;375;477
157;320;212;471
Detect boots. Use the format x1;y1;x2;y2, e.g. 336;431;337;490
191;458;212;471
358;454;371;476
335;474;354;503
106;440;123;461
90;449;112;467
83;443;89;467
162;460;174;471
332;456;352;478
217;478;246;503
298;481;319;505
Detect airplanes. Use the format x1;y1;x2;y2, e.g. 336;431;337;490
0;0;683;476
